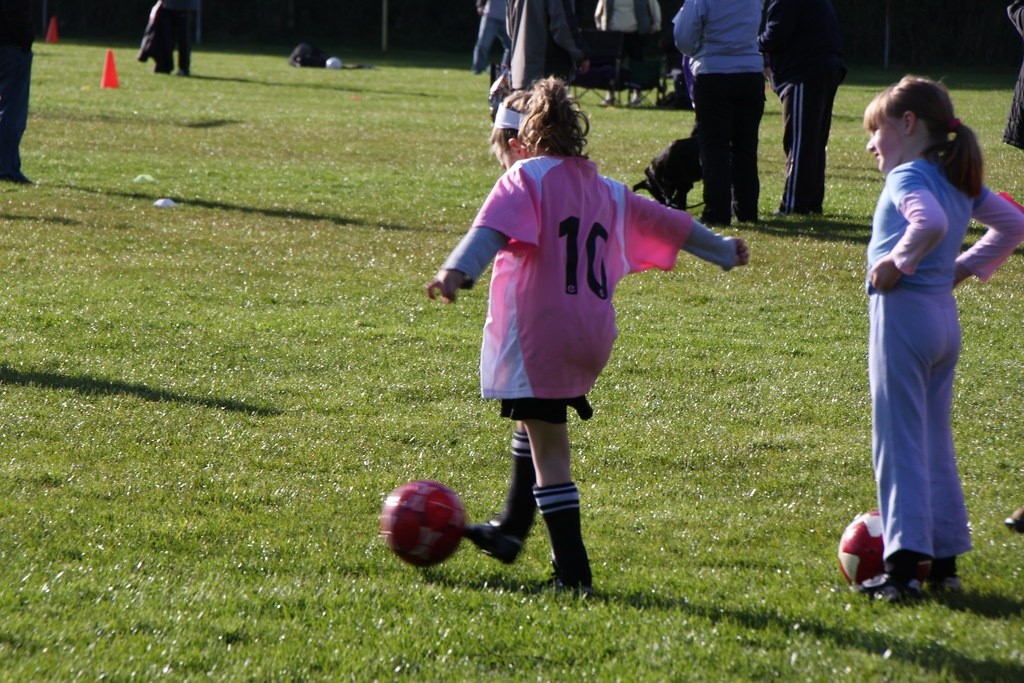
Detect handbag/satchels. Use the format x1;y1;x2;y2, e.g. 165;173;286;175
488;69;511;108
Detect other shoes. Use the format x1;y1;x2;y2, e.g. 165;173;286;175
0;170;30;182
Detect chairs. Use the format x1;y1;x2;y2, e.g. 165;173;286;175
617;26;672;109
569;27;622;105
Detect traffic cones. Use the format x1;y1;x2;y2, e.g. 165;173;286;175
99;49;120;88
45;17;59;44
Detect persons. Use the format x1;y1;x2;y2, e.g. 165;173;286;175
1001;0;1024;148
138;0;198;76
459;0;590;121
423;77;752;601
759;0;846;214
672;0;768;225
849;77;1024;608
595;0;662;108
1003;506;1024;532
0;0;42;187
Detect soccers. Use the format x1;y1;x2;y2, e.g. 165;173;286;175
837;510;932;586
326;57;342;69
379;480;466;568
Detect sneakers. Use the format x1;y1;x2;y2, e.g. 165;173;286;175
909;561;959;597
540;560;592;594
464;521;523;564
850;571;923;603
